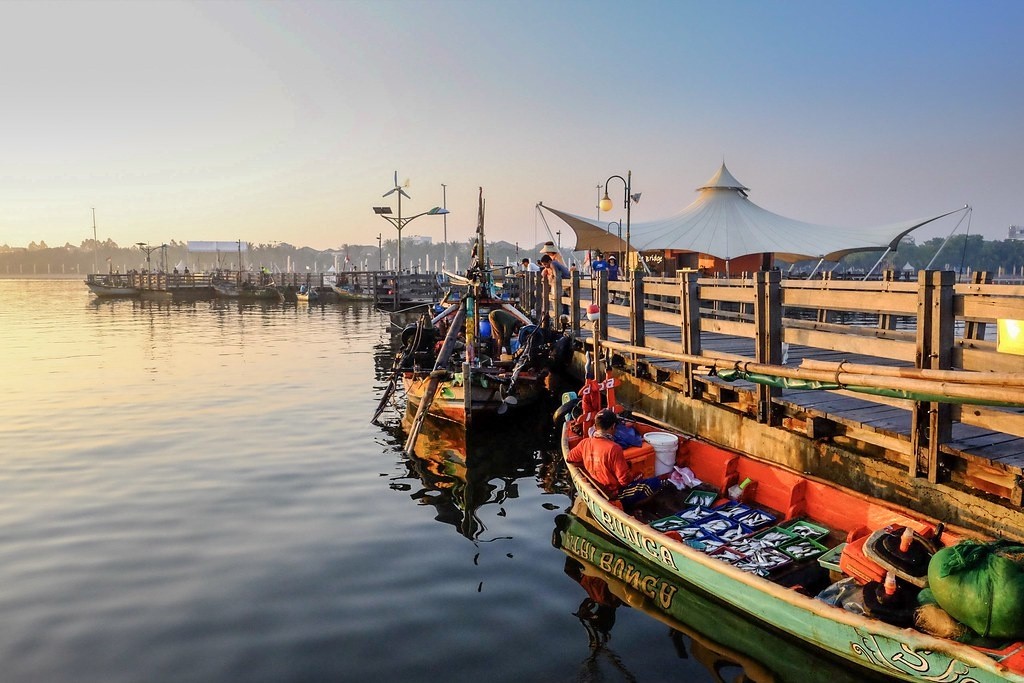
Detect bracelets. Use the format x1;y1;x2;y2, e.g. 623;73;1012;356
521;274;523;276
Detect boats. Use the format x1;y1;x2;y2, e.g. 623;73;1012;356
402;277;541;431
85;268;401;310
559;392;1024;682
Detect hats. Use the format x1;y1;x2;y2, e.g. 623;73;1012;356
540;242;558;253
595;408;619;425
609;256;616;260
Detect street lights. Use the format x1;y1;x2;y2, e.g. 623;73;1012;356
599;169;631;283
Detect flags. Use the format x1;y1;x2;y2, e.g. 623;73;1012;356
344;255;350;264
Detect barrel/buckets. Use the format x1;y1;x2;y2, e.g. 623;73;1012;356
643;431;679;477
453;293;460;300
478;317;492;337
503;293;508;300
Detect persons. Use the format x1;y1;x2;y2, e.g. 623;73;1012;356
515;241;577;320
566;407;660;508
488;309;528;358
591;254;608;274
605;255;624;304
105;263;408;277
